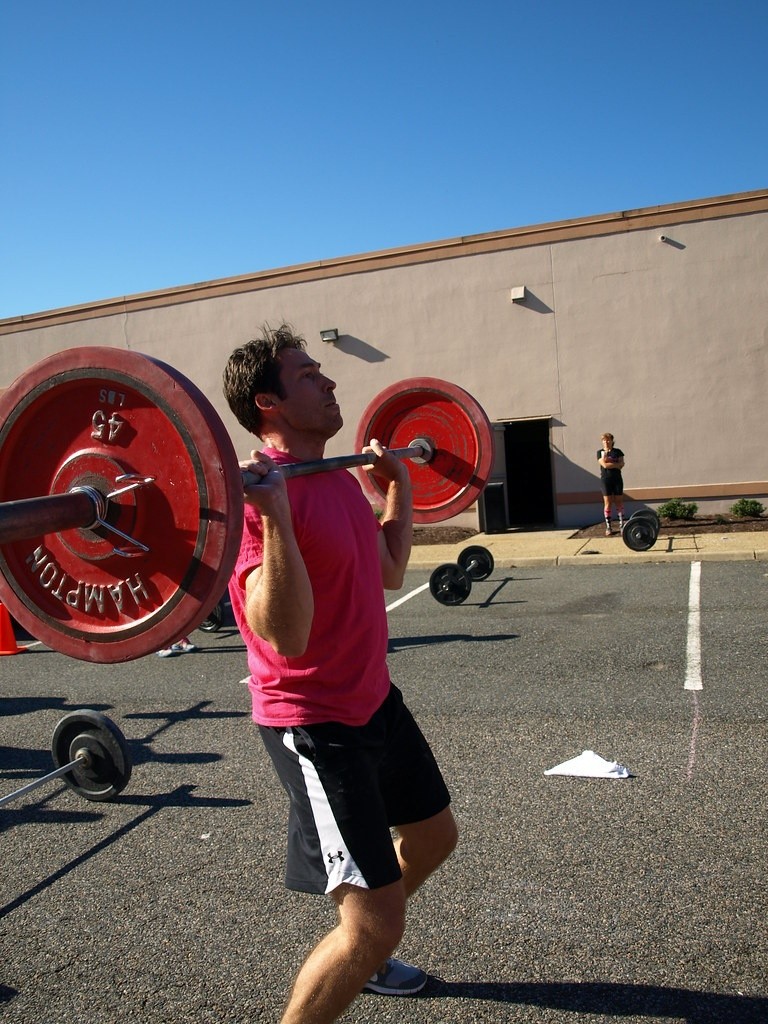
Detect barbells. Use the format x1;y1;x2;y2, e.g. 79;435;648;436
622;508;660;551
0;344;493;663
198;600;231;633
0;708;134;809
429;544;494;606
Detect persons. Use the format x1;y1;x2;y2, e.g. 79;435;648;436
228;320;457;1024
597;433;625;536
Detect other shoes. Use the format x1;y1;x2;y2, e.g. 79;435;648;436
157;647;173;658
171;637;196;652
359;959;430;997
620;526;623;534
605;529;613;535
